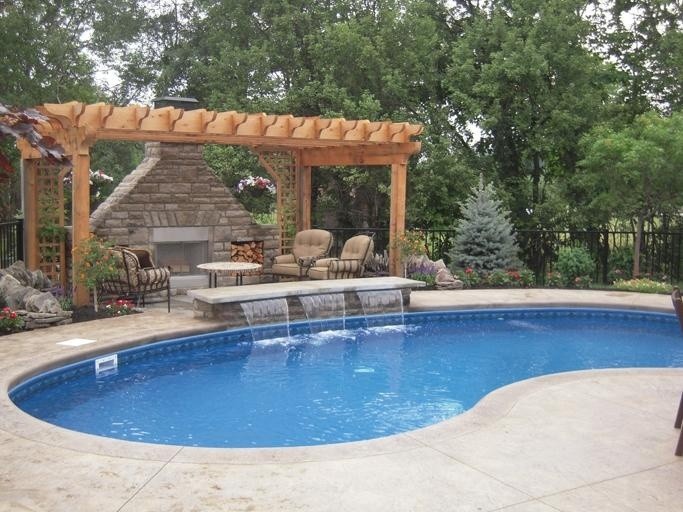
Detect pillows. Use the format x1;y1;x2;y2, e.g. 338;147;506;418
123;247;156;269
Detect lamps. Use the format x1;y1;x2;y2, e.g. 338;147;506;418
231;240;264;284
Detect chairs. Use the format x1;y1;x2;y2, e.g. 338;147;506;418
270;228;377;283
92;243;172;314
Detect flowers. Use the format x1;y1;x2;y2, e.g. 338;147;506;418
235;176;275;198
63;167;116;192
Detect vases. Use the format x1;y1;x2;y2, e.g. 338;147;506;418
249;189;265;199
87;184;100;195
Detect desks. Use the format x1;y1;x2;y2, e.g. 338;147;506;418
196;261;263;288
